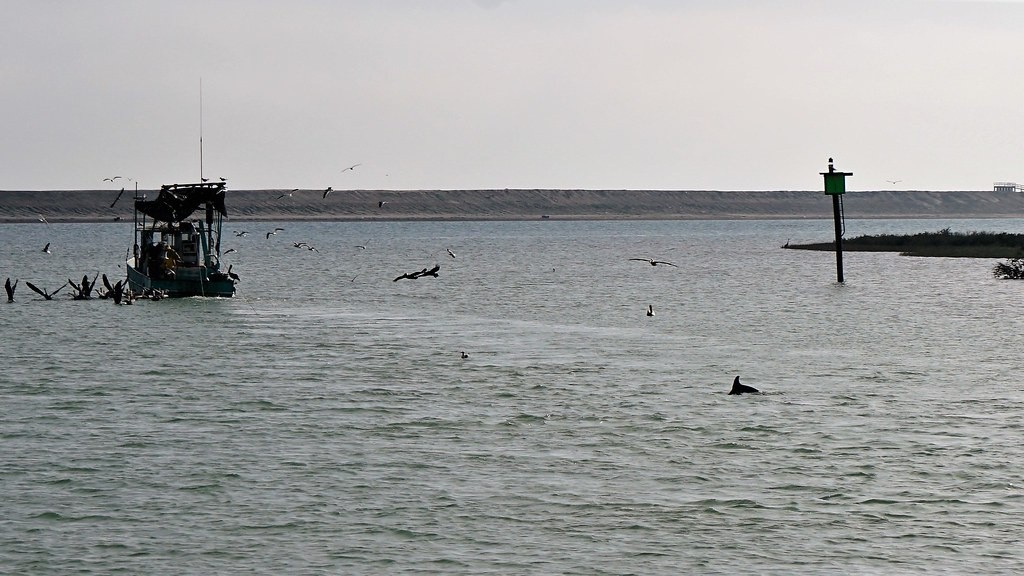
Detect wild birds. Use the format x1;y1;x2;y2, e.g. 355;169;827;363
5;177;457;307
628;258;679;268
646;304;656;316
460;351;471;358
341;164;363;173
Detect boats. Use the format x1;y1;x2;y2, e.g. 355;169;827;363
126;76;237;297
